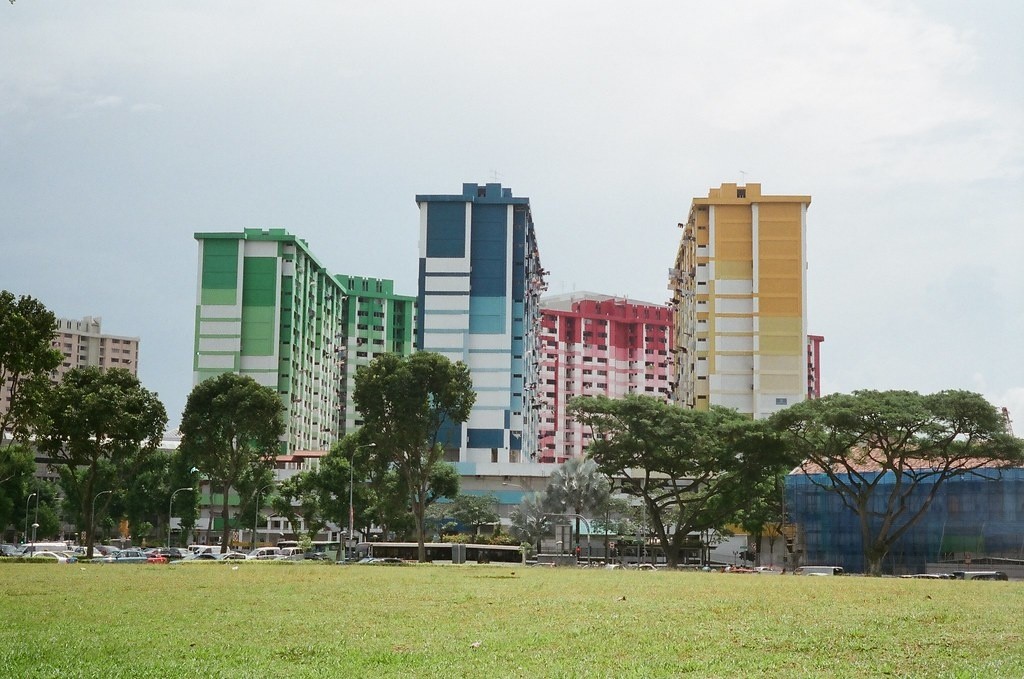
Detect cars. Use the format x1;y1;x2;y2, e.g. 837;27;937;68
900;571;1008;581
605;564;845;576
0;541;599;568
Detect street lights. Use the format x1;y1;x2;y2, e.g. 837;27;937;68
348;443;377;560
24;493;37;544
502;483;526;499
253;483;283;551
168;487;193;550
91;490;113;541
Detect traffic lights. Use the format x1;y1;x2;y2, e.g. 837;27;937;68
576;548;580;558
